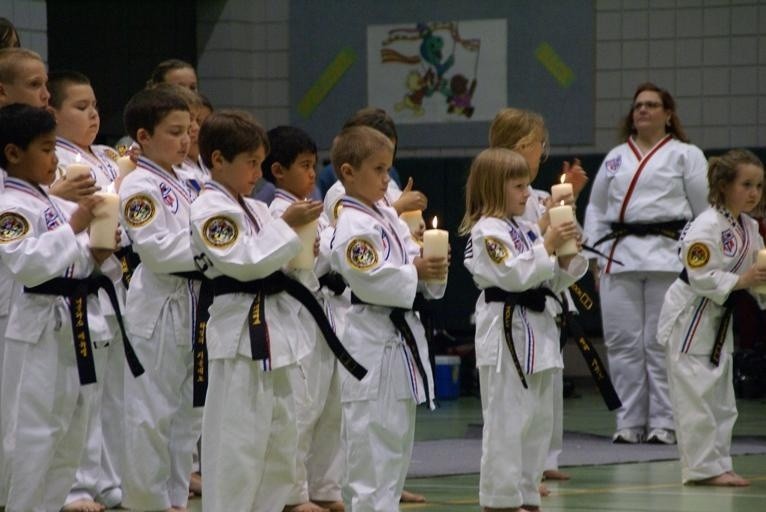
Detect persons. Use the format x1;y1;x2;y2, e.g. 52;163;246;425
113;60;212;512
581;83;713;445
488;107;588;497
655;147;766;488
47;70;142;512
0;17;20;49
458;146;583;511
0;104;122;512
0;47;107;512
191;108;451;512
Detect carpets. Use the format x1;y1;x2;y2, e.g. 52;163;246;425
406;423;766;479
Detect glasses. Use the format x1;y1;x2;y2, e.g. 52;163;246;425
613;427;645;444
629;101;664;111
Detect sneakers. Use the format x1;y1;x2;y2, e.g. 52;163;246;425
646;428;676;444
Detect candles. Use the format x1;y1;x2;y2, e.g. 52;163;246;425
422;216;449;284
88;181;120;250
399;210;424;247
287;198;315;271
65;152;90;182
751;250;766;295
549;199;579;258
551;171;576;212
117;146;137;177
